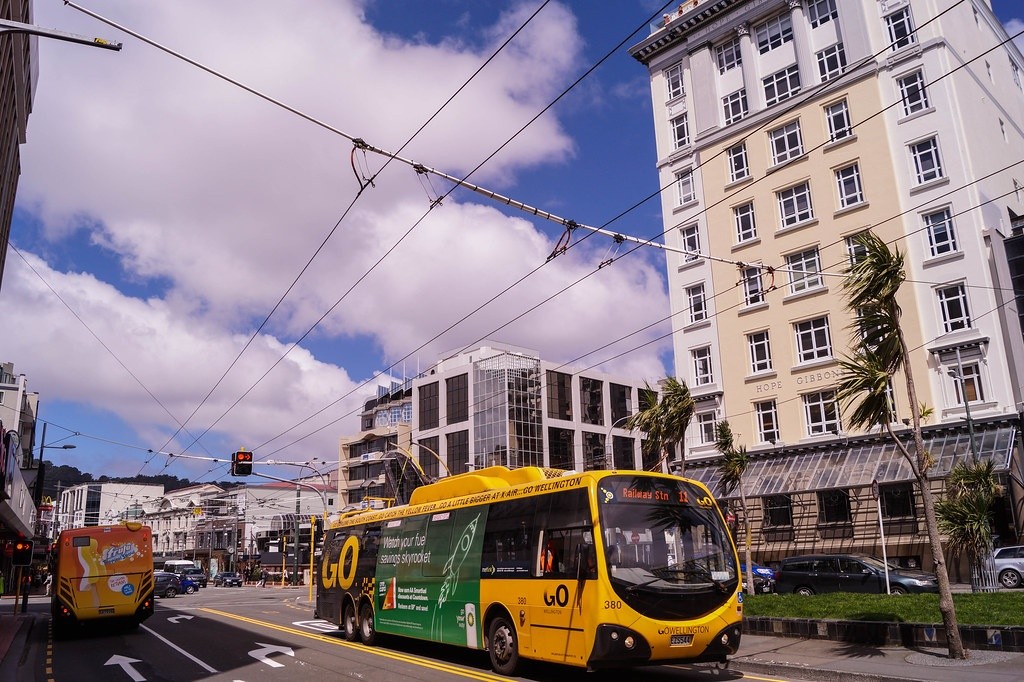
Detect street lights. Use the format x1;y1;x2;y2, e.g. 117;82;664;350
465;463;485;469
948;370;983;483
606;415;635;470
21;445;75;612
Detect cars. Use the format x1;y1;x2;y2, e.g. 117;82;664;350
152;572;179;598
741;572;774;595
181;568;207;588
741;564;775;581
972;545;1024;589
177;578;198;595
212;572;242;588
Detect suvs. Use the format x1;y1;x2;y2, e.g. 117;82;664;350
775;552;940;596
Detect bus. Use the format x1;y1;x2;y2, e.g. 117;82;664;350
52;522;154;635
315;466;745;676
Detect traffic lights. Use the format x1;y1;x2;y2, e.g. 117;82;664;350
13;541;33;565
236;452;251;474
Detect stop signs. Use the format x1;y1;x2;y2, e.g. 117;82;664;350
632;534;639;545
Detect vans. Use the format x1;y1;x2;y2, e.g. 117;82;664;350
153;557;169;570
164;560;195;578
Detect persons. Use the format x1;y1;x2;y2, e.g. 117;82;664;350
42;572;52;597
255;567;267;590
244;566;251;585
